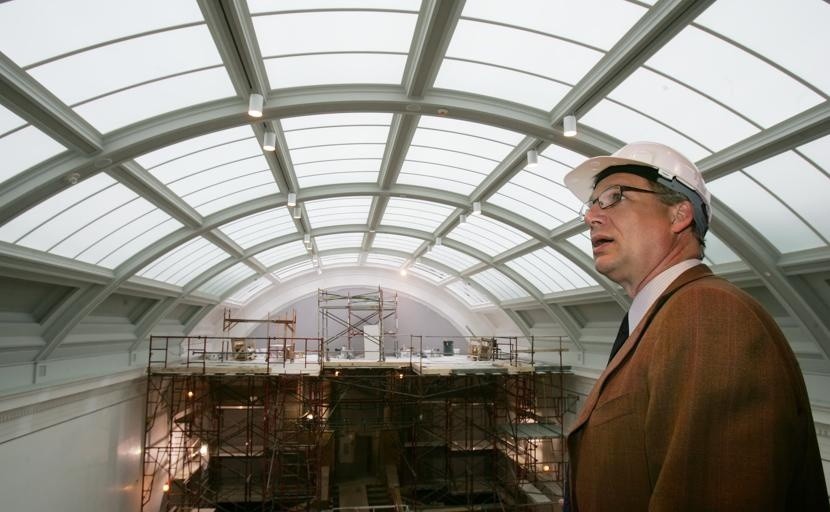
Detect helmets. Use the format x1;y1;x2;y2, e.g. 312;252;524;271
562;140;713;228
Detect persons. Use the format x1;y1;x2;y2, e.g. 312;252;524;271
559;140;828;510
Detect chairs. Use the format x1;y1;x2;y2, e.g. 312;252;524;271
487;339;501;359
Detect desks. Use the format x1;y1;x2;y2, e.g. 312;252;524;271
466;338;493;361
266;345;291;360
231;337;256;360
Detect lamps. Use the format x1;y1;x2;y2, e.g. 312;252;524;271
248;94;319;267
413;114;577;263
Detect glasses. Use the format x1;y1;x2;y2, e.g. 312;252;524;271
577;184;675;223
